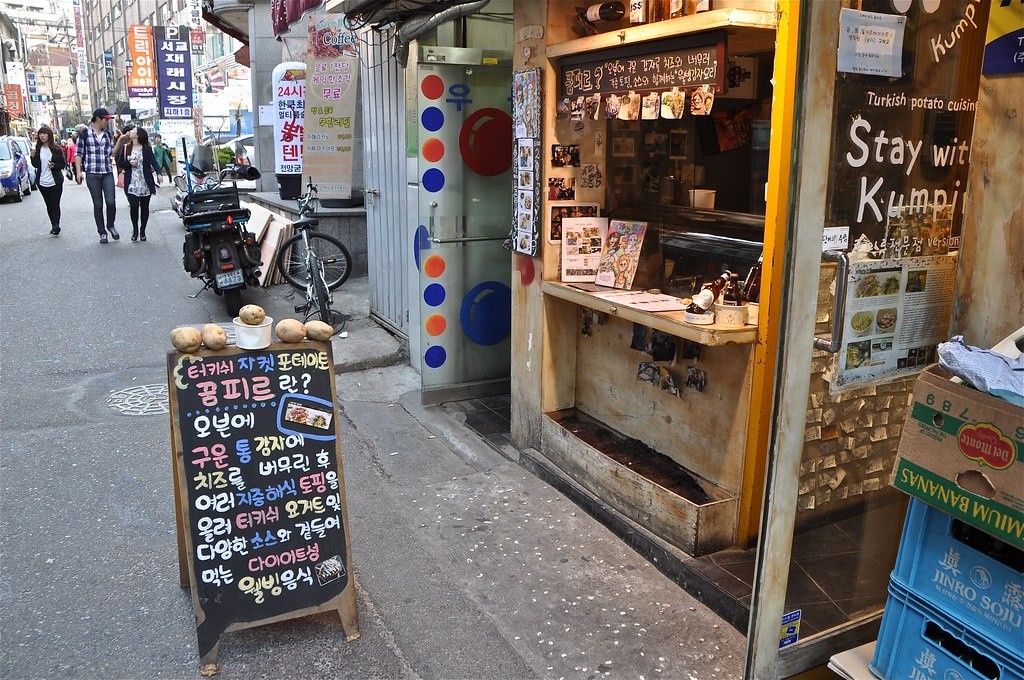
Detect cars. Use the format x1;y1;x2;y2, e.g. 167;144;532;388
0;134;40;203
219;134;256;180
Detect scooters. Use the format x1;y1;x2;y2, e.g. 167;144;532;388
171;160;264;318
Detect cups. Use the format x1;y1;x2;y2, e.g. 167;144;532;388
233;316;273;349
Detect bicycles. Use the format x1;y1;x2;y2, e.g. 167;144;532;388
277;175;354;330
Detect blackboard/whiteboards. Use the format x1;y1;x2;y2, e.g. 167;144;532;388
165;341;358;633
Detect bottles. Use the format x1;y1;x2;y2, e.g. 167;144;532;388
741;252;763;302
723;273;741;306
686;270;731;314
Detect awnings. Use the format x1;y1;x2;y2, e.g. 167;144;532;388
107;102;131;114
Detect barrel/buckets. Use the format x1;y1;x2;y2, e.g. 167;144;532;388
689;189;716;209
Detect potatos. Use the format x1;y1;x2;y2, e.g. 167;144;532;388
239;304;264;325
276;318;336;343
170;323;226;352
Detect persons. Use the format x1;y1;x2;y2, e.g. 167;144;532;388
29;127;66;235
61;108;173;244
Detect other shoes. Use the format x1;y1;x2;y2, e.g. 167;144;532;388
107;226;119;240
168;182;173;187
140;228;146;241
99;230;108;243
50;228;53;233
52;225;61;235
155;183;160;188
131;230;138;241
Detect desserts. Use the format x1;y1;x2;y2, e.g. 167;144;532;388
556;90;712;118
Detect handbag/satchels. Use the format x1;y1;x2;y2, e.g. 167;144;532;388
66;169;73;180
162;143;173;162
117;172;125;188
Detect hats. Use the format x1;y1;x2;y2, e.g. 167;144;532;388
93;108;114;119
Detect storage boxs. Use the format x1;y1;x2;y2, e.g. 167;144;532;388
868;359;1024;551
889;496;1024;659
868;579;1024;680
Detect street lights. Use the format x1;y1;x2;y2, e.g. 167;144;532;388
69;62;83;124
